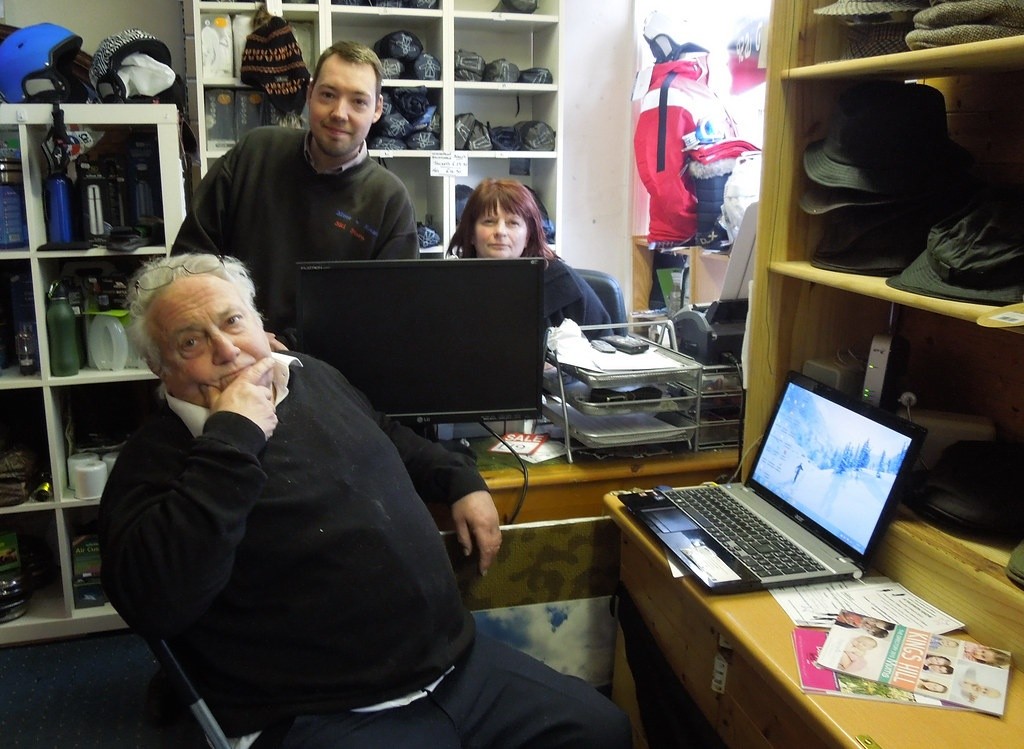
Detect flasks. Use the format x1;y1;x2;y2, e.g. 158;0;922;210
48;165;75;244
86;185;105;235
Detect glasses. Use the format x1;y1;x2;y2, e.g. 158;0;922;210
135;254;227;297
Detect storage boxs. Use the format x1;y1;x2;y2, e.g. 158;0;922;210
73;534;104;578
72;577;111;610
200;13;312;153
0;530;21;571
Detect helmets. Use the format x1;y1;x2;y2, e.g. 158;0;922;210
0;22;83;103
89;28;173;103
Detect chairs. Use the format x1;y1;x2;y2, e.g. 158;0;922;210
95;560;233;748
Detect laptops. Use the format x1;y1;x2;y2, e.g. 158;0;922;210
618;370;928;595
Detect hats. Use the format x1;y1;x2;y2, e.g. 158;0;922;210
800;83;1024;306
813;0;1024;60
241;6;311;117
908;437;1024;531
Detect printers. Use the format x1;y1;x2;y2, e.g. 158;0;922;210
669;297;751;366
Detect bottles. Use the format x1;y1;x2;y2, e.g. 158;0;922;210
136;164;154;220
17;332;37;376
47;285;79;377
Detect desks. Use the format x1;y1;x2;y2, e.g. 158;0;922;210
602;487;1024;749
439;446;739;666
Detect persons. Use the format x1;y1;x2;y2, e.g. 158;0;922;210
925;654;951;666
842;610;895;630
446;178;615;343
952;680;1002;700
170;40;420;352
962;642;1011;670
929;634;959;649
838;636;877;670
835;615;888;638
923;665;954;675
97;254;634;749
918;678;948;693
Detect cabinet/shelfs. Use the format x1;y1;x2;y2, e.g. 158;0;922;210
634;1;1024;668
0;102;191;644
182;0;562;255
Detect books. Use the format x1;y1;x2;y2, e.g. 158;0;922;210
790;608;1012;718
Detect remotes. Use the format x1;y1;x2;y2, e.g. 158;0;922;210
592;339;616;354
601;335;649;354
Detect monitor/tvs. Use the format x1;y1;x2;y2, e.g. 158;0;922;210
295;256;546;422
